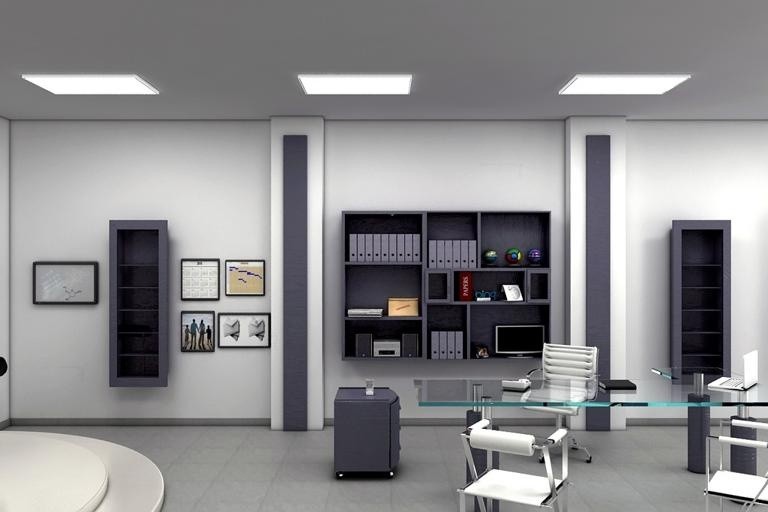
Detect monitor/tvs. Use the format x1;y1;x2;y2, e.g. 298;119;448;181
495;325;545;359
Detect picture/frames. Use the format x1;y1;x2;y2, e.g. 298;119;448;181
218;312;271;349
180;311;216;353
225;260;265;296
32;262;99;305
179;258;220;301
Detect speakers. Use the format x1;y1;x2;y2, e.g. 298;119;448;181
356;333;372;357
402;334;419;357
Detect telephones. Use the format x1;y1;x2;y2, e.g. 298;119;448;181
502;379;531;392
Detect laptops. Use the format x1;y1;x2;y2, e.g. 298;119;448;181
708;350;758;390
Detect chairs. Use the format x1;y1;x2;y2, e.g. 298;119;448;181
455;419;570;512
521;343;600;463
704;419;768;512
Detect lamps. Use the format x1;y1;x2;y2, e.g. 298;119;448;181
21;72;160;96
298;72;414;96
558;72;692;97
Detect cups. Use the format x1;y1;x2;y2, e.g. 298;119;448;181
365;378;376;397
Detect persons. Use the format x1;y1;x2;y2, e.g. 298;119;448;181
185;319;212;349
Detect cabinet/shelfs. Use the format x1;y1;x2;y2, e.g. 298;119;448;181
341;211;553;358
108;220;169;388
333;387;401;477
672;218;732;386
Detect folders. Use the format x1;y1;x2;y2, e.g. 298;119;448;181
430;331;464;360
429;240;476;269
349;233;421;262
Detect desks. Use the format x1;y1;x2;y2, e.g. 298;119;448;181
415;366;768;505
